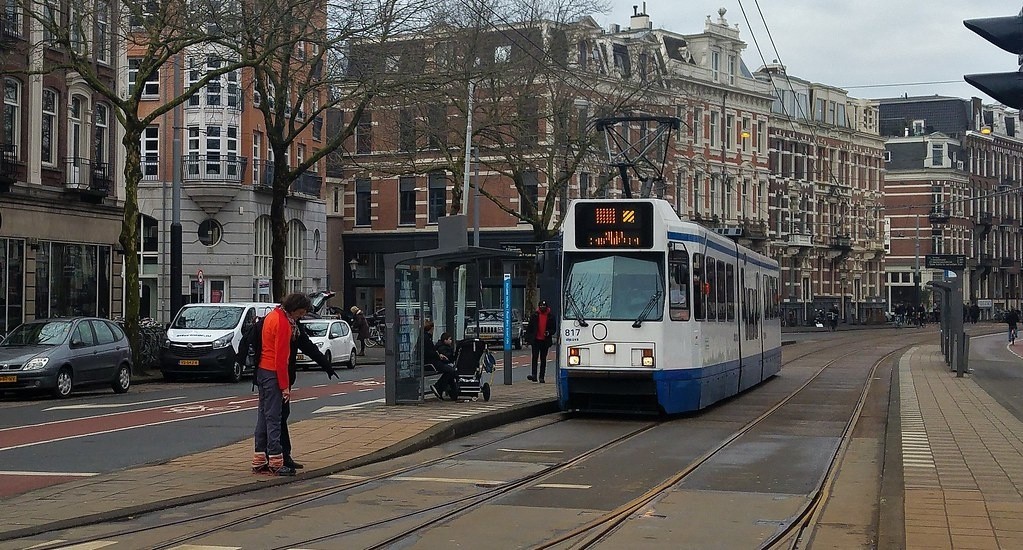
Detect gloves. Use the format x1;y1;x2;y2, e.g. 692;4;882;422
327;369;339;380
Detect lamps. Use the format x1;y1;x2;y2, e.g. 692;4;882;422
349;260;358;280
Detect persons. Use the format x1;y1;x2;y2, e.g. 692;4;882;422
351;306;370;356
906;304;913;316
435;332;454;364
895;303;905;319
963;302;980;324
251;291;339;476
1006;308;1019;342
832;305;839;323
417;320;454;400
917;304;925;312
525;299;556;383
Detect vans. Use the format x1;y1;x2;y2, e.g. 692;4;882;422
160;302;282;383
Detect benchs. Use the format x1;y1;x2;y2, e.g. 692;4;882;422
412;364;439;376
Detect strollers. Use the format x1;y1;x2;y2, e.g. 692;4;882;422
449;339;497;401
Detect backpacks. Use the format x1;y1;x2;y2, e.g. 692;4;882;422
242;316;265;368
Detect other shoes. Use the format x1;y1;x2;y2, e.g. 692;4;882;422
357;352;364;356
283;456;304;469
527;375;537;382
539;378;545;383
430;385;443;400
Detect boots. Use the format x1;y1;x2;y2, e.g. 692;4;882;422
252;452;269;474
269;454;296;476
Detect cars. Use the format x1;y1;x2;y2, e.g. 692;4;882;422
295;319;356;369
0;316;132;400
297;290;336;320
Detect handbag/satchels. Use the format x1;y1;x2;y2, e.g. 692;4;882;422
484;353;496;373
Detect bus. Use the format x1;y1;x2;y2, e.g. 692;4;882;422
534;115;781;414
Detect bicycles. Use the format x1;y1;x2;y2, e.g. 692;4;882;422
884;311;940;329
364;308;387;348
105;315;166;369
1009;325;1018;345
814;309;826;328
827;312;839;332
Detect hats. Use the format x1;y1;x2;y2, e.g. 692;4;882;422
539;300;548;307
424;319;434;332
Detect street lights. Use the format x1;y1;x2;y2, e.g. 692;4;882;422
348;259;360;332
457;73;486;340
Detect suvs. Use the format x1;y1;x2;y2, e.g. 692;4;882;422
465;308;524;350
